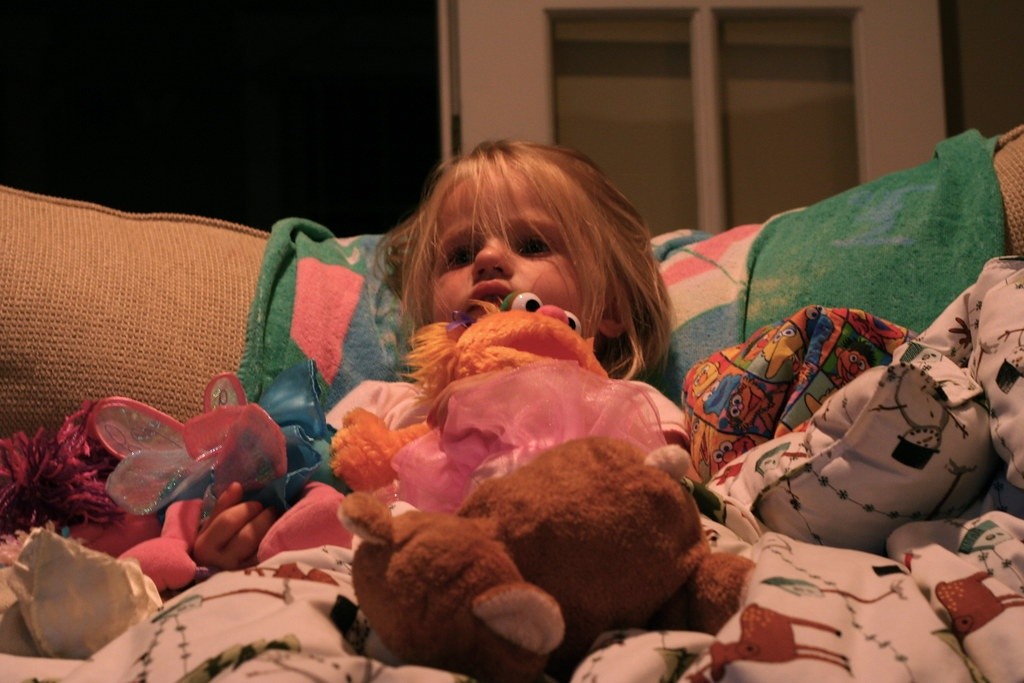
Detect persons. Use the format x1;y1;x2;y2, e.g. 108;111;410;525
187;138;704;572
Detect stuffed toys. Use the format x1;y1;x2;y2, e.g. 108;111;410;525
318;295;754;683
67;373;353;594
